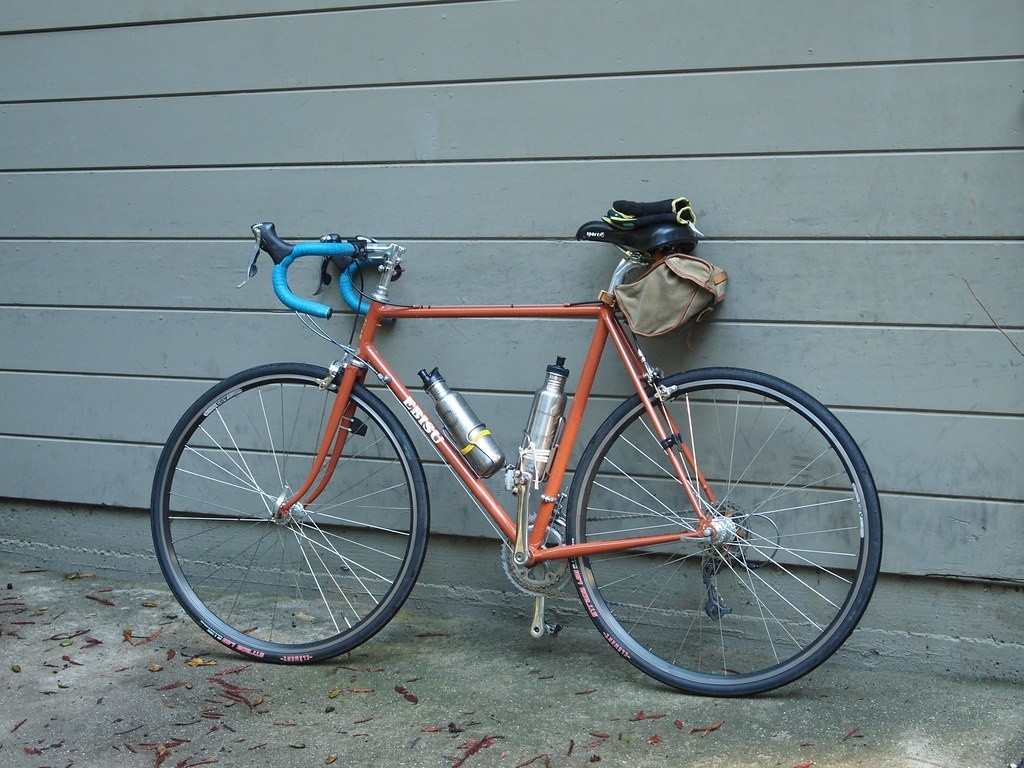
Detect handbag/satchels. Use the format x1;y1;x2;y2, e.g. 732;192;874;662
613;253;727;353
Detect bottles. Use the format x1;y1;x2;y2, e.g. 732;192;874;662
515;355;570;481
417;367;506;476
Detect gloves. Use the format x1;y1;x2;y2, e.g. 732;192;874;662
602;197;696;229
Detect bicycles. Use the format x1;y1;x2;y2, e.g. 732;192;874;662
150;220;886;699
